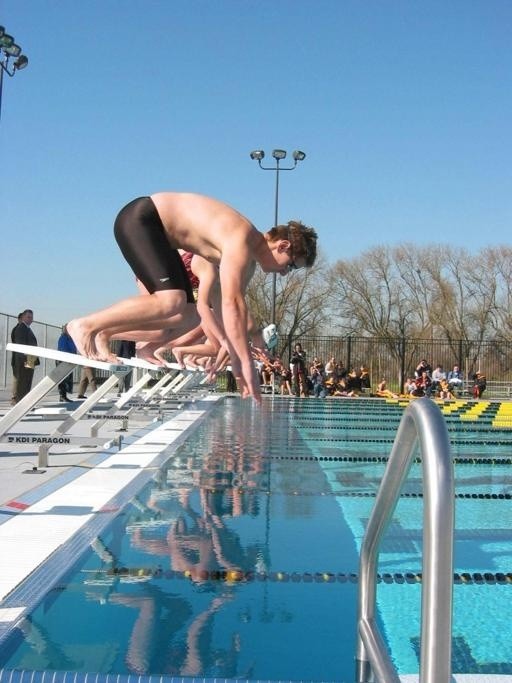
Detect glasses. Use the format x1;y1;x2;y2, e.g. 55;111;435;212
287;230;296;270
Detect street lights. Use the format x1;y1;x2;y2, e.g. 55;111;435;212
250;145;306;361
1;25;28;123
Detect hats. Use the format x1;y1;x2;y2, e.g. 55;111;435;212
263;324;278;348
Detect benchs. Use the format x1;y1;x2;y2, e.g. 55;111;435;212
436;379;512;396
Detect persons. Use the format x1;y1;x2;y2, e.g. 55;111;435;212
56;323;77;403
252;348;293;395
11;310;40;406
225;365;236;393
405;360;464;400
66;191;316;406
77;366;98;399
375;378;399;399
116;340;136;397
471;370;486;399
290;343;309;398
306;356;372;398
